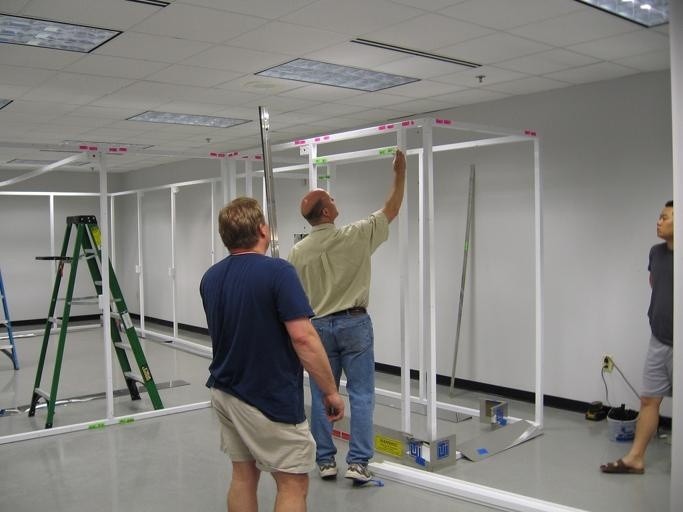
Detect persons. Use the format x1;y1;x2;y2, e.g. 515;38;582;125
600;200;673;474
288;151;406;481
198;198;345;512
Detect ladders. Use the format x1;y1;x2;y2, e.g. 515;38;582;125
28;214;164;430
0;271;19;371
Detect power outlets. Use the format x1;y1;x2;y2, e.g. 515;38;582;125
602;354;614;372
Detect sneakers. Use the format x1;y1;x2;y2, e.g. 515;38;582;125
316;456;339;481
342;461;374;484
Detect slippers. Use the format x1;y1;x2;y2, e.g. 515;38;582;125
600;459;645;476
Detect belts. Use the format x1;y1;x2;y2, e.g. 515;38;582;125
327;307;366;317
